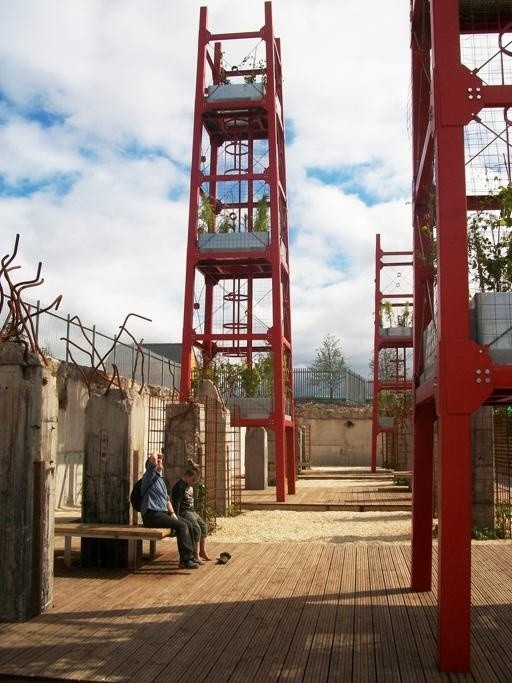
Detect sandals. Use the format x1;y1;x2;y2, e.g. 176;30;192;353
199;553;212;561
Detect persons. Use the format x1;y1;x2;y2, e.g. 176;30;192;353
140;452;205;568
173;467;212;560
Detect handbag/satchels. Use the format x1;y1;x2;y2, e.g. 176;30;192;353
131;475;158;511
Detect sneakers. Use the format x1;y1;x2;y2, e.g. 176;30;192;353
179;558;206;568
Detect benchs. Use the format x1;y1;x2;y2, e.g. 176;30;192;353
54;514;174;570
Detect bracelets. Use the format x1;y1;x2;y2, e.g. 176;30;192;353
170;512;175;514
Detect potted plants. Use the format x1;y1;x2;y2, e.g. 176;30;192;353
191;346;274;419
380;299;413;337
467;165;512;363
198;191;270;253
377;393;399;429
